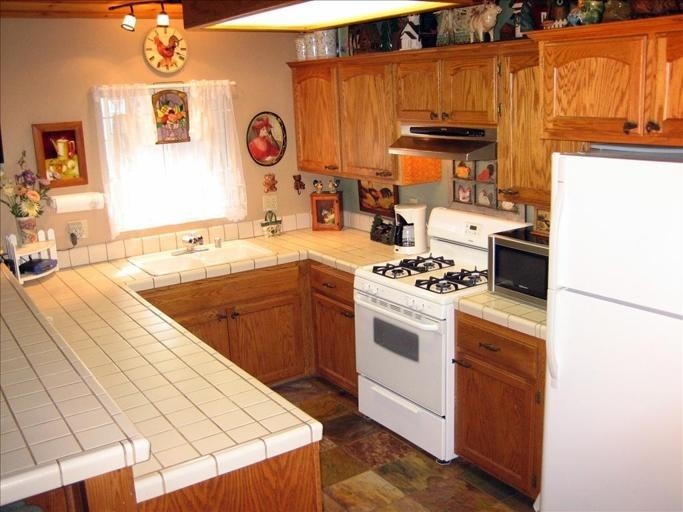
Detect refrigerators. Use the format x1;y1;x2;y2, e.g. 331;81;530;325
538;149;681;512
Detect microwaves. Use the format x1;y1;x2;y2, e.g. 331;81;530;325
486;229;548;310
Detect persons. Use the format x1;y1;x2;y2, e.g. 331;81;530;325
248;116;280;162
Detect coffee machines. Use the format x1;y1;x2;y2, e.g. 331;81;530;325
393;198;427;255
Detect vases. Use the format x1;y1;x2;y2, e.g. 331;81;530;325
10;218;49;249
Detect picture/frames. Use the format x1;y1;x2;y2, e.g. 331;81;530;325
33;118;87;190
537;28;678;147
497;44;582;208
356;182;398;214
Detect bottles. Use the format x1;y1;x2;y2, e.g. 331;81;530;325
294;31;337;63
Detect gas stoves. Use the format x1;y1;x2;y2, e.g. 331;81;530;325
355;253;487;303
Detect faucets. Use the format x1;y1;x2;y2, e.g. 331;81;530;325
182;234;205;250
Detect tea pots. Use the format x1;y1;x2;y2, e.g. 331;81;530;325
49;137;75;161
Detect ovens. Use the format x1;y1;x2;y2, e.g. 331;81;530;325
349;288;459;465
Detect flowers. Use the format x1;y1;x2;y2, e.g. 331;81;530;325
2;168;48;215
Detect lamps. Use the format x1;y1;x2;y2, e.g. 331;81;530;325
123;11;141;31
158;10;172;27
182;1;476;34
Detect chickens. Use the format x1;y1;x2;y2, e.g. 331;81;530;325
154;34;179;65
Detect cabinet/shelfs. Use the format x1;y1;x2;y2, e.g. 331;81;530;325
141;264;310;388
310;263;355;395
397;57;498;126
290;62;396;182
456;296;539;501
7;229;60;285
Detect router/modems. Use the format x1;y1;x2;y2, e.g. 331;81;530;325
20;256;58;273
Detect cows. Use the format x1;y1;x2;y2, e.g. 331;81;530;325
468;0;503;43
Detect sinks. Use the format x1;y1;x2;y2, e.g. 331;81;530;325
126;240;279;277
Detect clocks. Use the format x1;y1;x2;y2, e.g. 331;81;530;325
141;20;189;71
